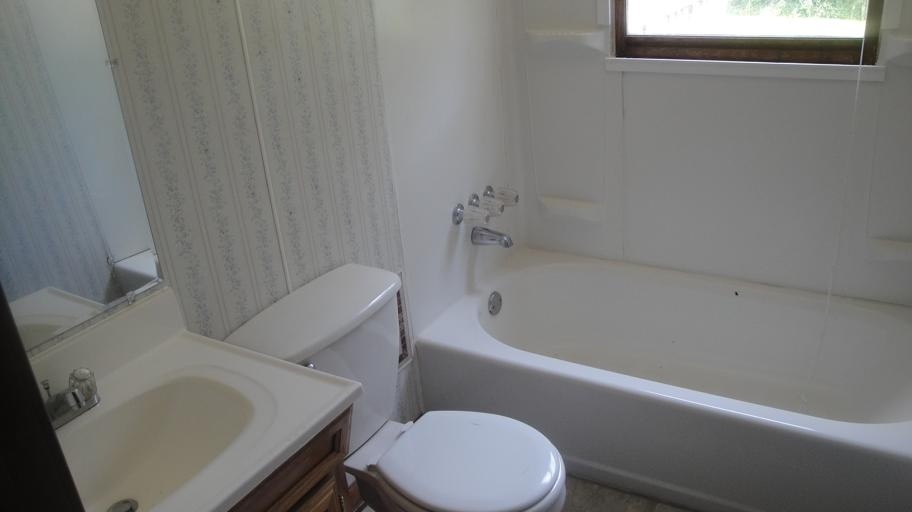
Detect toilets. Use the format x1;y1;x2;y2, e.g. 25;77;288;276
221;262;568;511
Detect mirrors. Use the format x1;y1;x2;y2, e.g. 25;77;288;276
0;1;166;360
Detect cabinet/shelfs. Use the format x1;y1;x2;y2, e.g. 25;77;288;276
229;405;354;512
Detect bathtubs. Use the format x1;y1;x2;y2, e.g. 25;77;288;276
414;245;912;512
112;248;159;295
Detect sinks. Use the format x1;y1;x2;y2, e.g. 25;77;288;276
33;289;363;512
8;284;104;353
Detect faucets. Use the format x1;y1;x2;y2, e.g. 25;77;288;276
471;225;514;248
40;366;102;430
